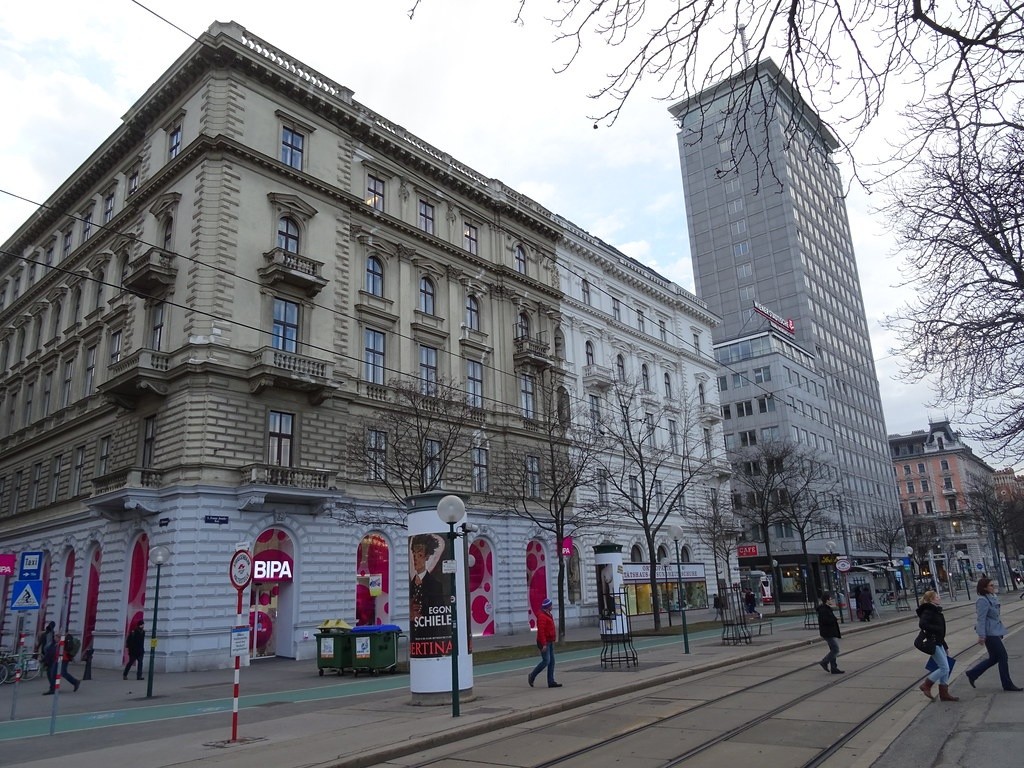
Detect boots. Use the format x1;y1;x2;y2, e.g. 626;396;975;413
920;677;935;701
938;684;960;701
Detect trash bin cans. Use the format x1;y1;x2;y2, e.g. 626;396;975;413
352;624;403;678
313;618;352;677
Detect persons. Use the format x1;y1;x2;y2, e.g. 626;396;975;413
817;594;845;674
859;588;873;622
528;599;562;688
744;588;762;619
914;591;959;701
123;621;145;680
966;578;1024;691
1020;592;1024;600
36;621;81;696
713;594;721;621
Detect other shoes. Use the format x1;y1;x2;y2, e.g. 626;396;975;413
966;671;976;689
73;679;80;692
759;614;762;619
137;676;145;680
123;673;127;680
548;682;562;687
820;659;831;673
831;668;846;675
42;691;55;695
528;673;536;688
1004;683;1024;690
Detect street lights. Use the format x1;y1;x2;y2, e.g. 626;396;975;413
667;524;691;654
147;545;171;697
825;541;845;623
956;551;972;601
904;546;919;607
436;494;478;716
661;557;671;626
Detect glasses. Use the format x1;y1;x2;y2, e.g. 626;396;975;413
984;584;994;587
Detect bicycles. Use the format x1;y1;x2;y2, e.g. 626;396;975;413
0;648;43;684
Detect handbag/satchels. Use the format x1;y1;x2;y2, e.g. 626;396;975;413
914;628;936;655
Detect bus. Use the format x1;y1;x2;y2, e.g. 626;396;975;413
739;571;773;606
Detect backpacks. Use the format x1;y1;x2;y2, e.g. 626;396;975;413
70;638;81;657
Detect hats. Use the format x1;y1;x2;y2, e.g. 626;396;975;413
542;598;552;608
